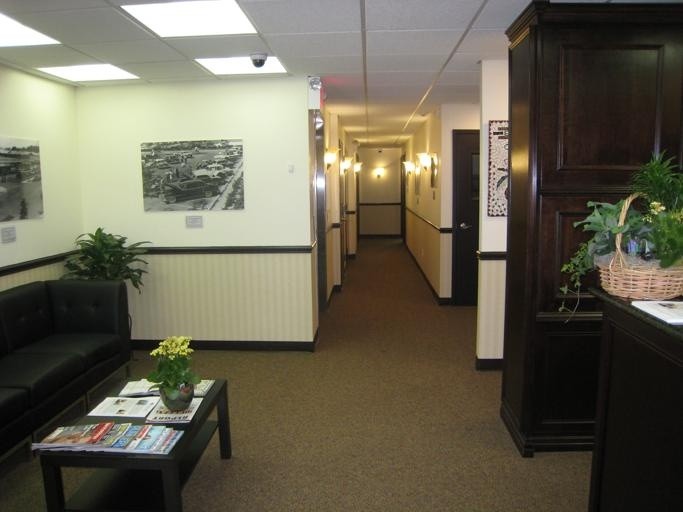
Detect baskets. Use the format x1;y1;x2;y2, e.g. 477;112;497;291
595;192;682;300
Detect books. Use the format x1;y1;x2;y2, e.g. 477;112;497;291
118;380;216;395
630;300;683;326
146;397;204;423
30;421;185;455
87;395;160;418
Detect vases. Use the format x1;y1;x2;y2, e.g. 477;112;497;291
159;382;194;411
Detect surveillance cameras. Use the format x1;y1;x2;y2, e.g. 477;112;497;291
250;53;268;68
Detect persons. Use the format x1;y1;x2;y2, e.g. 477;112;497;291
143;142;234;207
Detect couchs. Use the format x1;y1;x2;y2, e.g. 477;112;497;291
1;280;133;465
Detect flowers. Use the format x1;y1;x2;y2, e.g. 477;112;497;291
559;149;683;323
146;335;202;399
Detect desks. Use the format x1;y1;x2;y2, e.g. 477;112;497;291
582;286;681;511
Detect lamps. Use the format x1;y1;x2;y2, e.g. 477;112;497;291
417;151;438;188
324;146;340;171
